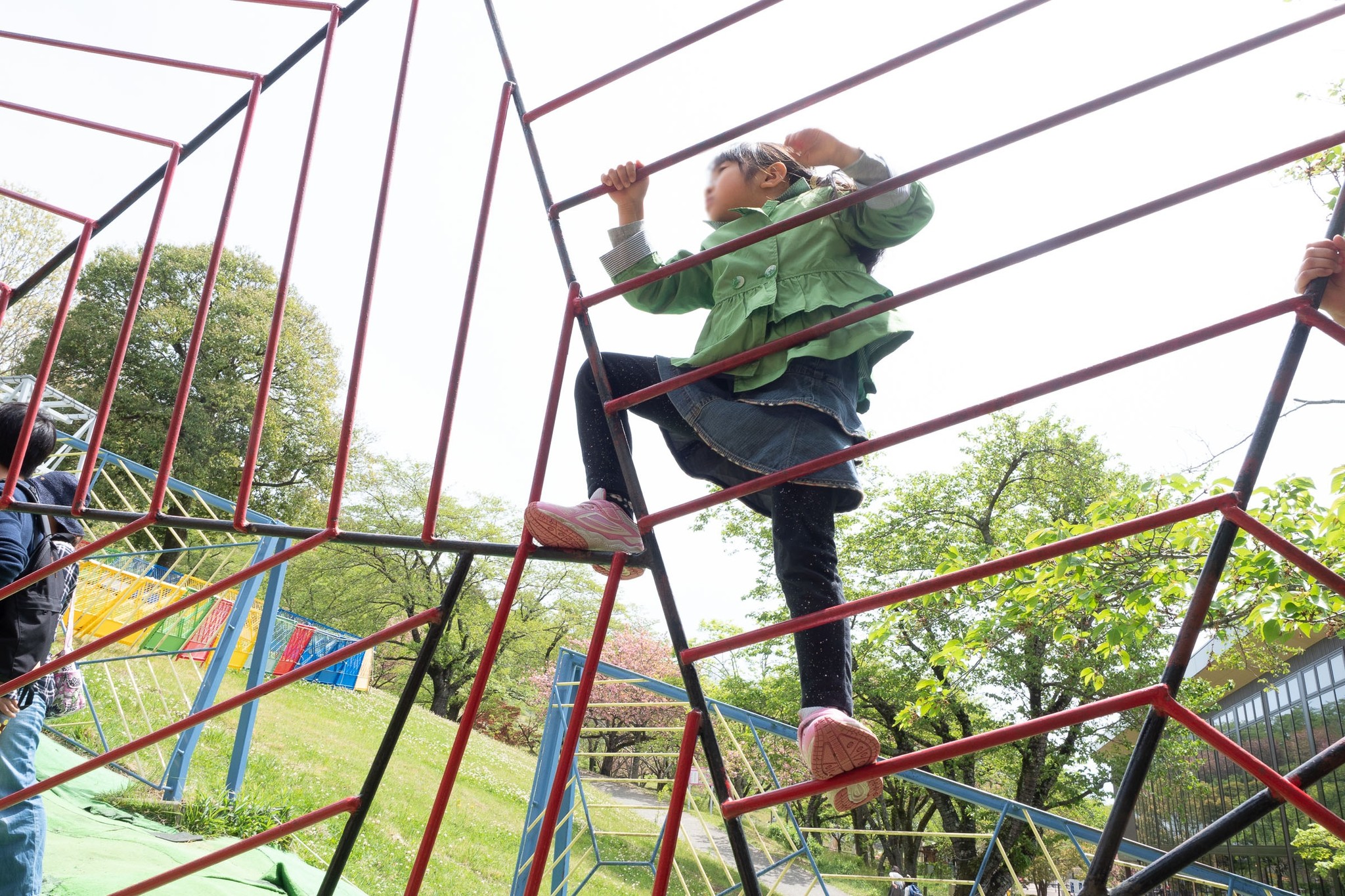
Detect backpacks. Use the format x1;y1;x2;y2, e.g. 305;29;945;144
0;478;82;712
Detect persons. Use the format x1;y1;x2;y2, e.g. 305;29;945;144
522;126;937;811
1068;891;1073;896
0;402;90;890
1295;230;1345;338
888;865;922;896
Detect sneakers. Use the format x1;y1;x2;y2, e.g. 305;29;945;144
523;486;647;580
796;706;884;814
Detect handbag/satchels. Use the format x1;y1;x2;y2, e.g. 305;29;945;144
43;648;86;720
